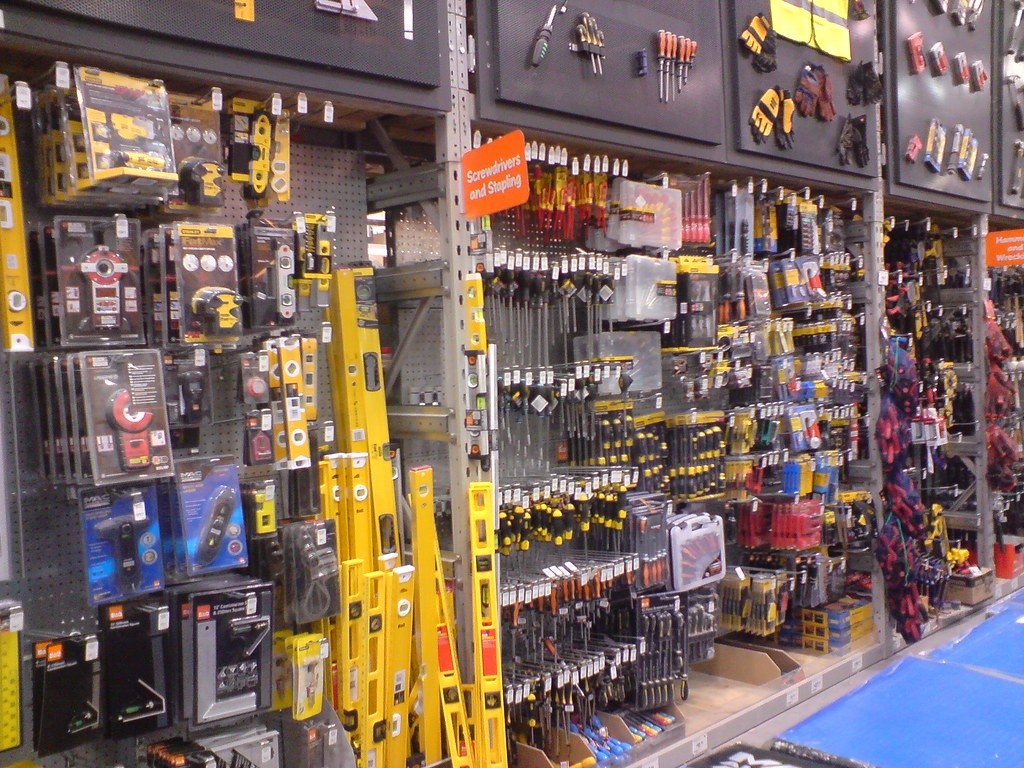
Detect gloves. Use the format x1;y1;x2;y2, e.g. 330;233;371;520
846;62;886;106
750;84;797;149
738;12;777;73
792;62;836;123
876;345;930;644
835;113;871;169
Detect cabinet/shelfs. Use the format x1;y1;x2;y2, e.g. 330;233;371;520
878;198;986;654
479;123;881;768
986;215;1024;601
0;27;476;766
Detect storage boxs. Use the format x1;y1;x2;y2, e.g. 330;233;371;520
775;633;872;657
777;619;876;644
946;567;994;606
778;599;874;629
994;534;1023;579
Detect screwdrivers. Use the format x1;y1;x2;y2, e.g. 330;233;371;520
484;241;732;768
